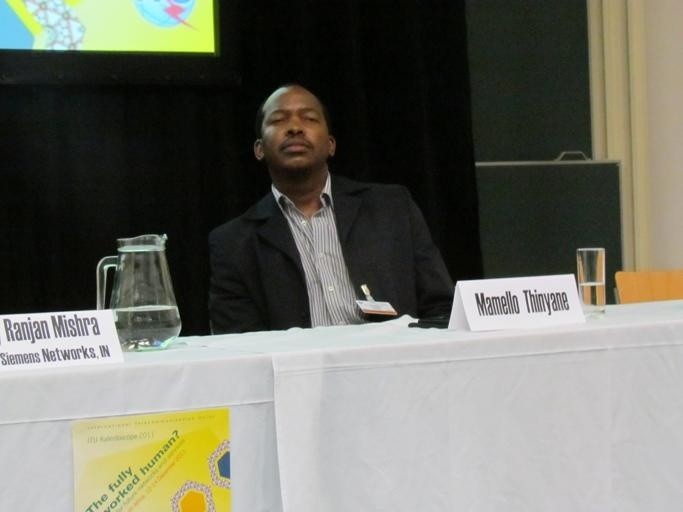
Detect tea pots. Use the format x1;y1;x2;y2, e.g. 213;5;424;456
95;230;185;352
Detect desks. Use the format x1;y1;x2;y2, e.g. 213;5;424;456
0;300;682;512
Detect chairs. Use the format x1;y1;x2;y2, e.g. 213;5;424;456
613;269;683;305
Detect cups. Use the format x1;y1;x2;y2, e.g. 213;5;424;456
574;248;607;314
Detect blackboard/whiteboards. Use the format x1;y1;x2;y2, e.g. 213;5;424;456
475;159;623;304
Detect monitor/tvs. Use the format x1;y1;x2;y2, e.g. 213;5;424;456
0;2;223;88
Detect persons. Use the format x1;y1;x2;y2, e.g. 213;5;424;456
204;83;454;333
